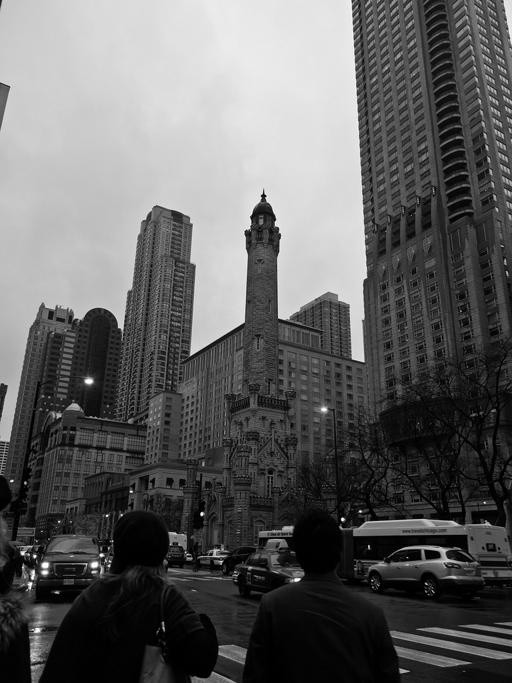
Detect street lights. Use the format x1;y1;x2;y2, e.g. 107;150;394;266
319;402;342;518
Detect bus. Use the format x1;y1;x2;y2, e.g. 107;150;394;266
258;518;512;598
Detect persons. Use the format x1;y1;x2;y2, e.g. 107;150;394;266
242;507;401;682
0;474;33;683
39;509;219;683
16;544;44;583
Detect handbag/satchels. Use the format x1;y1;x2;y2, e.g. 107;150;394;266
142;581;192;683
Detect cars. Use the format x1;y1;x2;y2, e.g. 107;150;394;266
11;533;116;601
